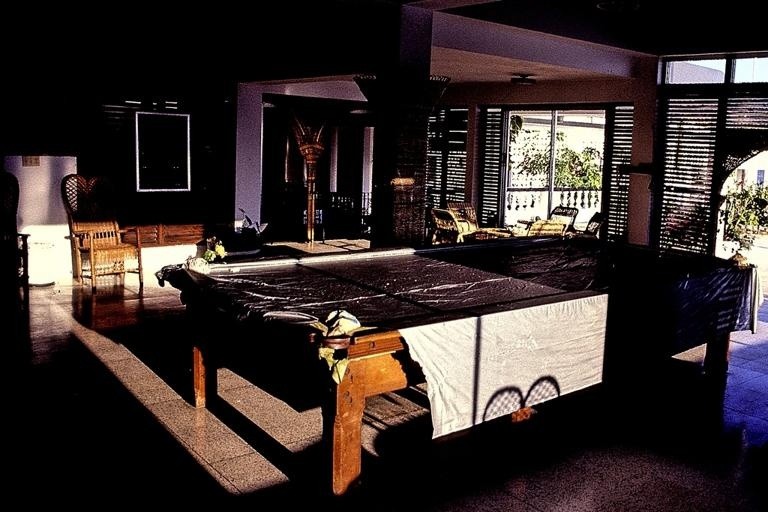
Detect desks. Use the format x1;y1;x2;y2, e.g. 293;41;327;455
728;260;765;330
165;229;756;499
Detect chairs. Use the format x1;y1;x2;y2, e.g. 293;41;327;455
423;201;613;246
57;171;147;295
292;190;389;244
0;171;32;307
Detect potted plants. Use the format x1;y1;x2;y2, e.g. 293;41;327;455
719;181;768;254
